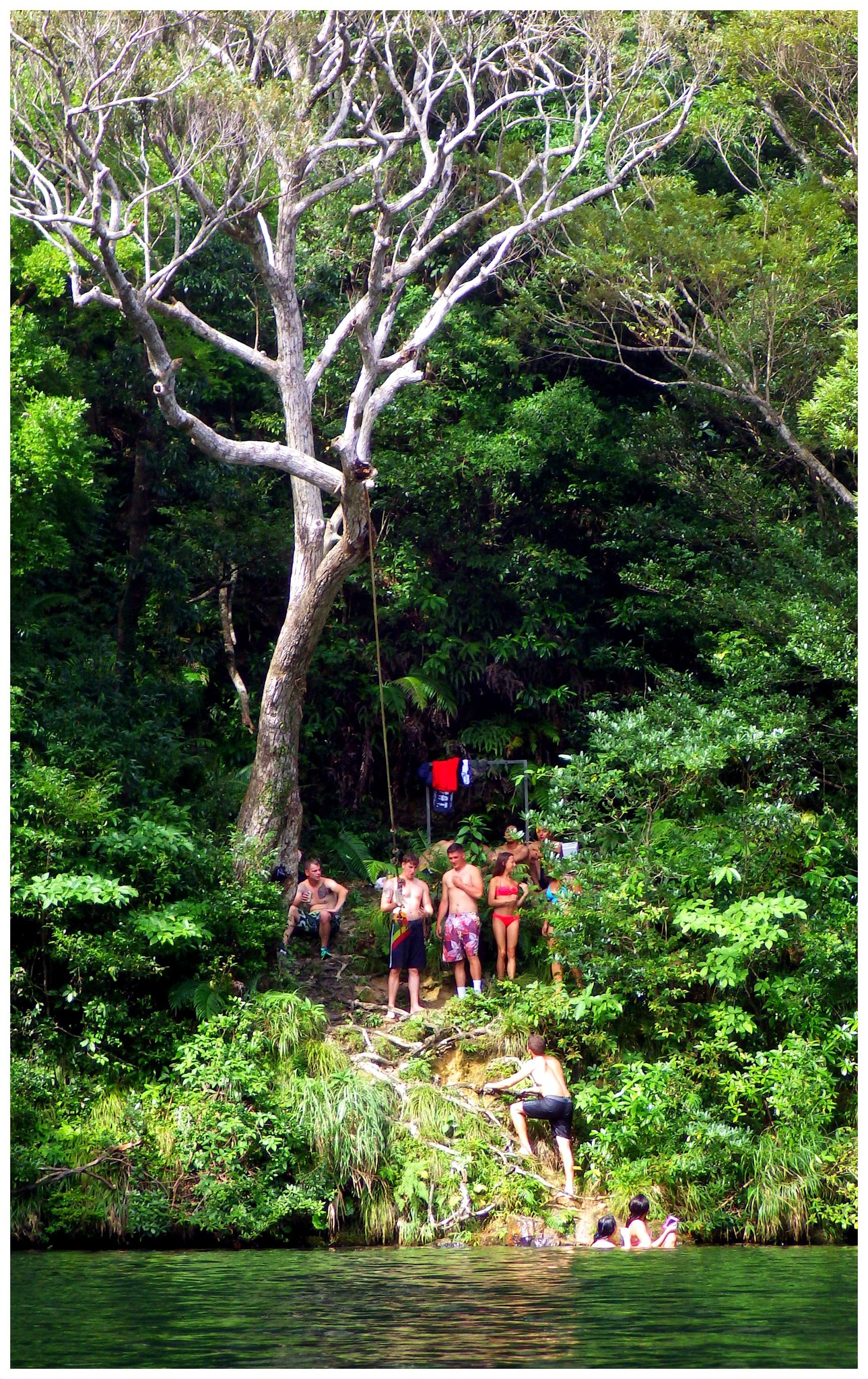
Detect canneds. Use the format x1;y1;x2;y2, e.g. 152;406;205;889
306;891;313;903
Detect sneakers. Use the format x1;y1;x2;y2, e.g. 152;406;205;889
281;950;288;957
321;947;332;960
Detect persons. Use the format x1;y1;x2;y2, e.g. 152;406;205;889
591;1215;632;1249
542;852;585;998
488;852;530;993
417;821;579;907
484;1035;576;1197
436;843;484;999
620;1194;677;1250
380;853;434;1020
276;859;348;960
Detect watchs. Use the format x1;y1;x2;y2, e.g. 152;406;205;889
427;913;430;918
